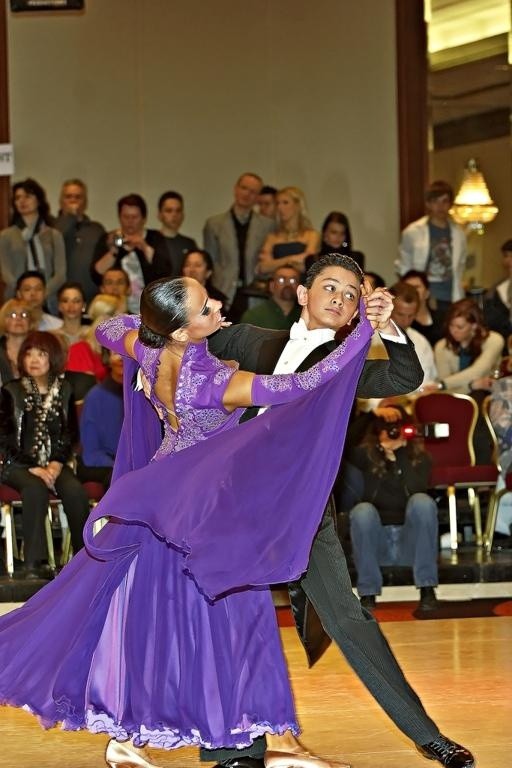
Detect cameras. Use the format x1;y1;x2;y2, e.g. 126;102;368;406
375;415;450;453
114;236;124;246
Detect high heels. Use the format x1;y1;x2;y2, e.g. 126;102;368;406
264;751;351;768
105;739;164;768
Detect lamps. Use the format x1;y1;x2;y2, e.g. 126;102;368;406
448;157;499;235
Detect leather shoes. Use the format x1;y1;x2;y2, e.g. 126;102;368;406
417;733;474;768
360;597;376;609
420;596;437;610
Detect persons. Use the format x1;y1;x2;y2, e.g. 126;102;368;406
339;400;443;614
93;272;393;768
1;167;512;580
196;253;482;768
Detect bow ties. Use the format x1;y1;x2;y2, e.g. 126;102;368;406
290;318;335;345
